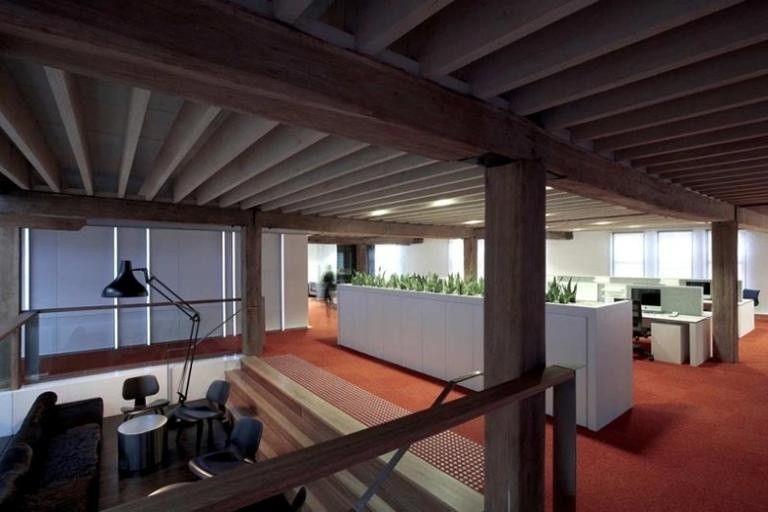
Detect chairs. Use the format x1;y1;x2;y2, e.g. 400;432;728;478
743;289;759;307
188;419;263;481
120;376;169;423
616;299;654;361
174;383;231;459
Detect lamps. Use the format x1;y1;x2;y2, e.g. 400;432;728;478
100;260;201;430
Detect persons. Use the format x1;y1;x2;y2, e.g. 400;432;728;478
321;263;337;320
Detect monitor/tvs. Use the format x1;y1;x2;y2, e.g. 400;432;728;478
548;276;743;317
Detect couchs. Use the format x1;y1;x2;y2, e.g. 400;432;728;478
0;392;102;512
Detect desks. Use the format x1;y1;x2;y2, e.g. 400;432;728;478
633;294;754;368
